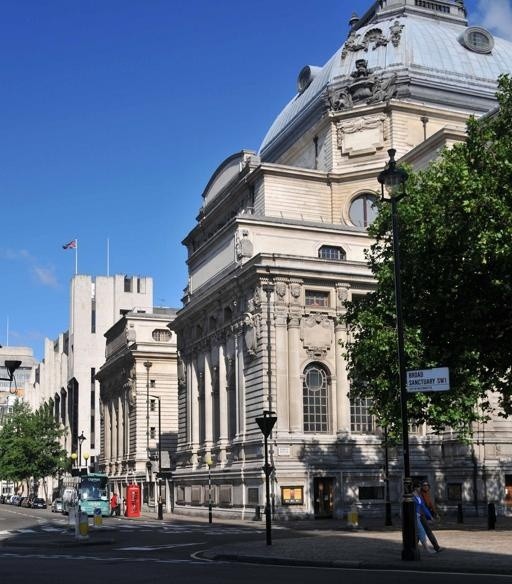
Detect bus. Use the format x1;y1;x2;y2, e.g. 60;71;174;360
61;473;111;518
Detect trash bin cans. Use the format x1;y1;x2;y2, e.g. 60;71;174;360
79;511;89;536
94;508;102;527
347;511;359;528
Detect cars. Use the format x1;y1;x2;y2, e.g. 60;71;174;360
0;493;47;510
50;497;65;513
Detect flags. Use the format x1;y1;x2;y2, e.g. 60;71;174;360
61;240;76;249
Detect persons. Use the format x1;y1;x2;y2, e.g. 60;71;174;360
411;482;437;554
110;492;121;517
416;480;447;553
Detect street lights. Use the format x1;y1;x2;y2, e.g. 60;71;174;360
130;391;163;520
206;460;214;523
0;343;23;381
379;146;419;562
71;429;89;476
